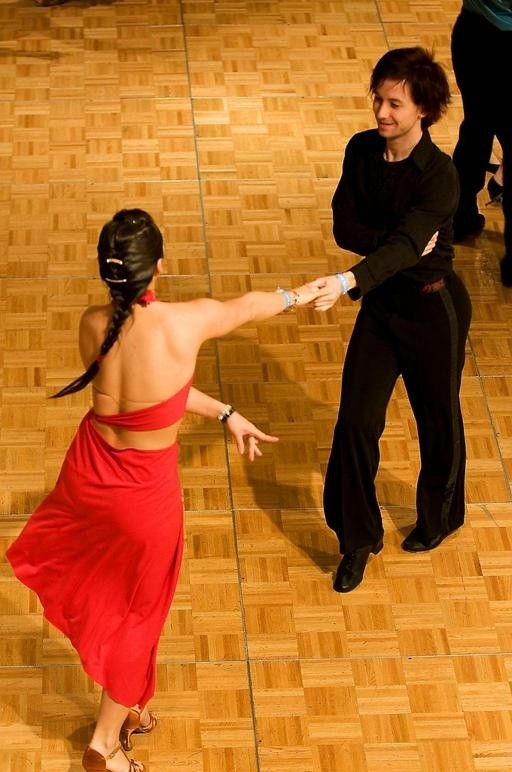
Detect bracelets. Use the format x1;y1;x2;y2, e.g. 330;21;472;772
336;274;348;295
276;288;291;307
220;407;236;424
217;404;232;422
282;287;303;305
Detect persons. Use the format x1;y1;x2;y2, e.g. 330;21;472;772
448;0;512;288
4;207;322;772
486;151;504;207
310;47;474;594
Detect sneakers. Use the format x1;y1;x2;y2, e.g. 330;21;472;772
454;215;485;246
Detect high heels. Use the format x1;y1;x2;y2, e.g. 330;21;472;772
80;741;146;772
120;704;158;752
332;537;382;593
400;525;452;550
